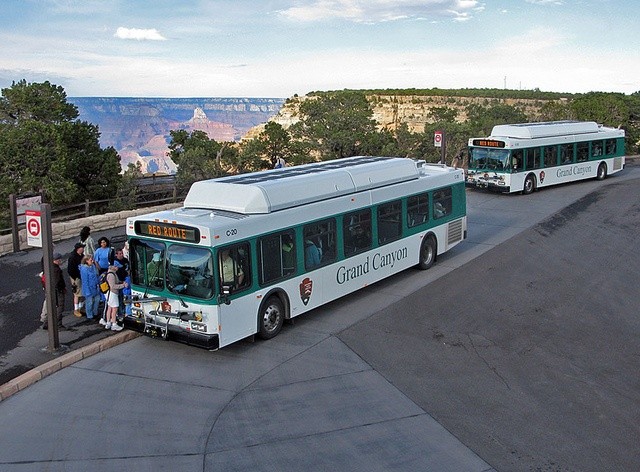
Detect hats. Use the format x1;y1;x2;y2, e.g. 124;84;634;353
114;260;123;268
74;243;86;250
52;253;62;260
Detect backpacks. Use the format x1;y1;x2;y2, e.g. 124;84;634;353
97;272;117;303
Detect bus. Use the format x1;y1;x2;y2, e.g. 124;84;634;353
455;120;625;193
108;155;467;351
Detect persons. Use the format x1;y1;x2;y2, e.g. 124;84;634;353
504;154;517;169
80;255;99;322
67;243;86;317
98;272;109;326
104;265;127;332
212;250;244;294
305;234;321;269
40;252;66;331
143;253;162;287
280;236;296;274
94;236;114;274
274;154;285;168
112;248;129;282
122;241;140;263
79;226;96;260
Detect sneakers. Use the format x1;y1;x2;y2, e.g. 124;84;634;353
111;325;124;331
56;325;70;331
74;310;83;317
99;318;107;325
